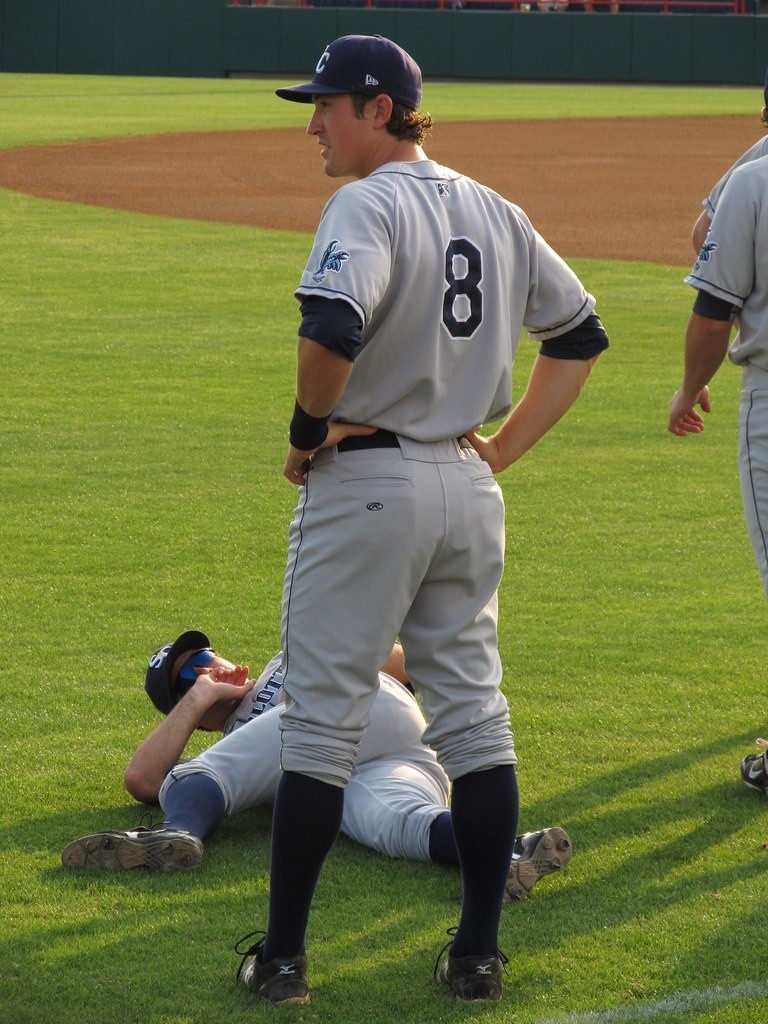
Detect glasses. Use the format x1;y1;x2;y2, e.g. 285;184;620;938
173;648;217;703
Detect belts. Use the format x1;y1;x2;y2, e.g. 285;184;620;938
337;428;473;453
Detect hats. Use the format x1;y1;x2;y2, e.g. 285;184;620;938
145;631;210;716
275;34;423;111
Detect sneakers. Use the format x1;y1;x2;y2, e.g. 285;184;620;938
434;927;509;1003
62;811;204;873
235;931;311;1005
503;827;573;902
741;738;768;798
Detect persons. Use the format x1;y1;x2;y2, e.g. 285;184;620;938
236;36;612;1002
62;633;572;902
667;84;768;794
